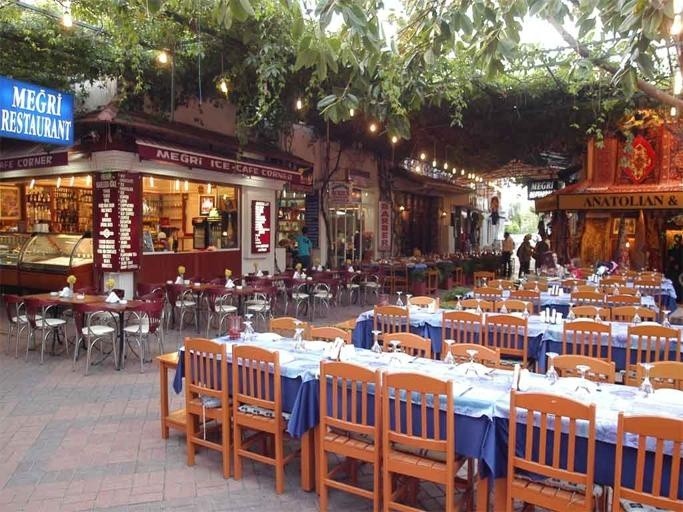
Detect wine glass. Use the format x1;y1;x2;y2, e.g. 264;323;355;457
573;361;592;396
637;359;654;398
369;328;383;363
288;320;309;355
466;347;481;382
242;313;256;347
544;352;560;386
442;339;457;369
244;263;668;325
387;340;402;368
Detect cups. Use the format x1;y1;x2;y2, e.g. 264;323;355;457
173;240;178;253
184;279;190;286
227;316;241;339
168;238;173;250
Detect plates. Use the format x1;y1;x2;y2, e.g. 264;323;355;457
654;386;683;404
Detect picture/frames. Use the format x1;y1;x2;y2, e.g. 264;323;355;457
199;195;214;216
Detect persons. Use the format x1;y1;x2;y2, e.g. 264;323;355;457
297;227;313;270
502;232;515;272
517;232;548;279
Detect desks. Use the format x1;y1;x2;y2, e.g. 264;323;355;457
159;351;186;440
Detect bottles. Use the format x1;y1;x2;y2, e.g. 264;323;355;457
142;214;160;234
26;186;93;234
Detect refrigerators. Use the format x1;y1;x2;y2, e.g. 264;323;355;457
206;215;226;248
192;216;208;250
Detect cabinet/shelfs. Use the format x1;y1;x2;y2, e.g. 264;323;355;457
10;183;185;251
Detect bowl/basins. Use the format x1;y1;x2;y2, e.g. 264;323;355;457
76;295;85;300
166;280;173;285
118;300;128;305
194;282;201;287
237;286;243;290
50;291;58;297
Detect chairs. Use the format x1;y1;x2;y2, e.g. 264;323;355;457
320;360;381;512
232;344;300;494
185;337;233;478
507;389;596;512
613;411;683;511
158;252;683;390
5;275;157;377
383;371;467;512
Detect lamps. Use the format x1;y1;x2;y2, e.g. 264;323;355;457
349;109;355;117
392;136;397;143
461;168;465;176
421;152;426;161
63;8;72;27
296;99;302;110
392;203;405;215
468;168;484;182
444;142;449;170
220;50;227;91
159;52;167;62
370;124;376;133
453;167;456;174
437;212;446;221
432;137;437;168
